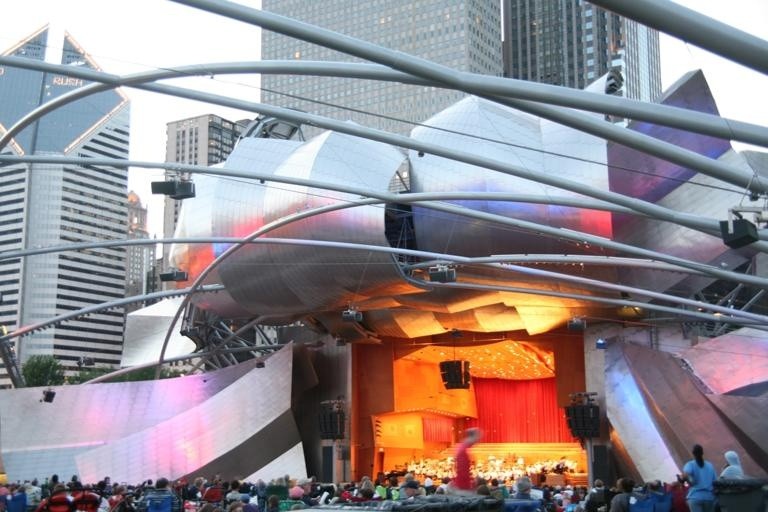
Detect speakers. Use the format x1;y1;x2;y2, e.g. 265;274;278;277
439;360;471;390
564;405;601;438
317;409;345;439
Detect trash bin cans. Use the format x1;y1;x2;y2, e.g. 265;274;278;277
714;478;768;512
